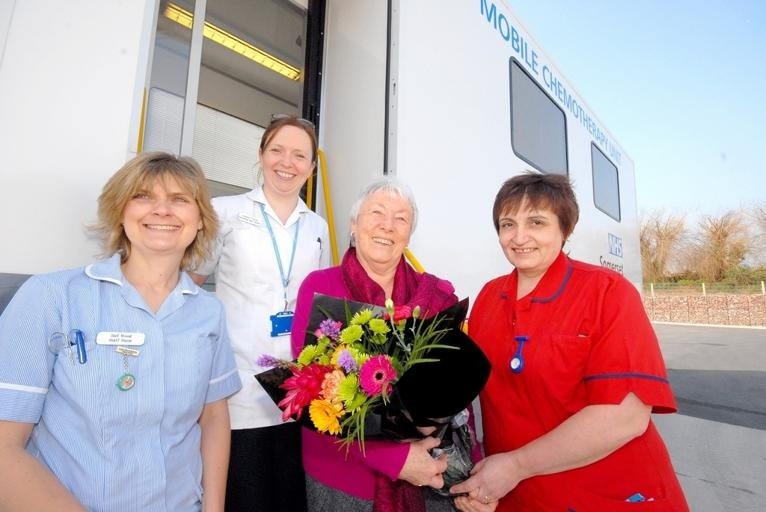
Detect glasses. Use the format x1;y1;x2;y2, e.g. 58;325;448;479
270;112;315;132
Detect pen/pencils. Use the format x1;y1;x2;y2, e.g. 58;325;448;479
76;330;87;364
317;237;321;249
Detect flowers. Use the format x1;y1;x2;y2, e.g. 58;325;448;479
256;299;491;458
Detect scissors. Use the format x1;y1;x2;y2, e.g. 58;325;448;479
48;328;85;355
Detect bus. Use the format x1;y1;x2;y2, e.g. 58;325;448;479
0;0;645;504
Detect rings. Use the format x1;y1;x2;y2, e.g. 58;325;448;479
480;494;493;503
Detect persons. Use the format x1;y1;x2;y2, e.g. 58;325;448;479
0;147;248;511
175;109;339;511
446;170;693;511
288;172;504;511
